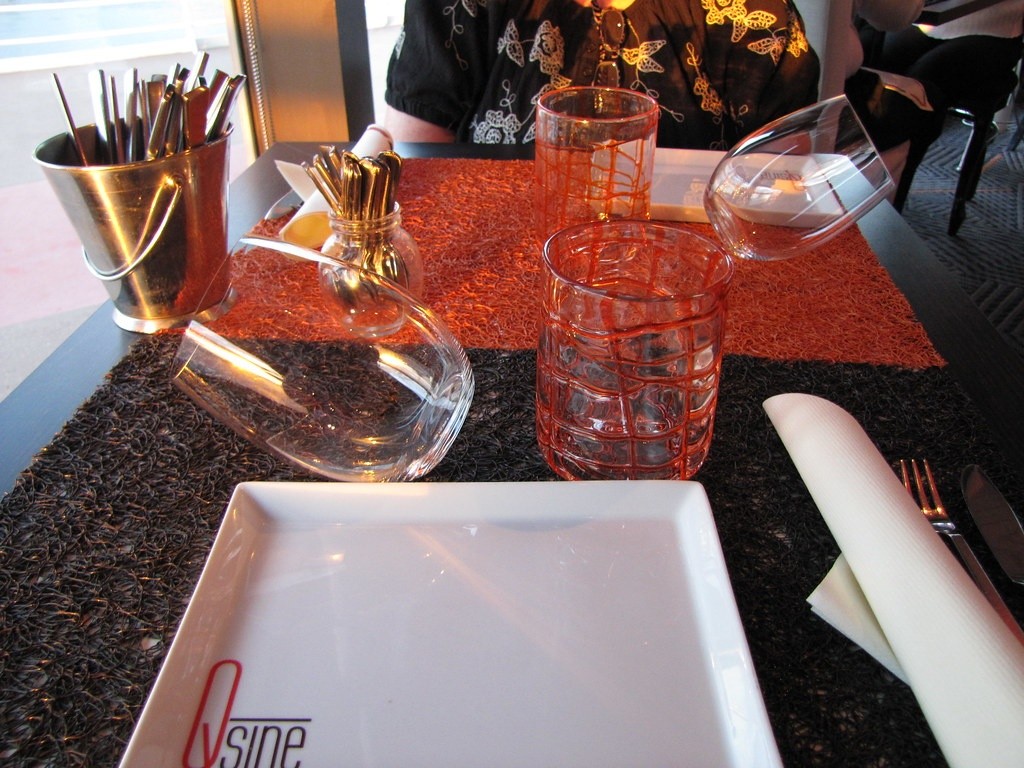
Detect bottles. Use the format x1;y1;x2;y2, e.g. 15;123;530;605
317;201;423;338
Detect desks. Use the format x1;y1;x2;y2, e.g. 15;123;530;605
2;137;1024;767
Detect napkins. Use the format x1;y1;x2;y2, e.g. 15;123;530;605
759;392;1022;766
272;120;396;248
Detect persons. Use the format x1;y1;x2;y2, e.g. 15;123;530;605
794;0;926;153
385;0;822;156
843;0;1024;149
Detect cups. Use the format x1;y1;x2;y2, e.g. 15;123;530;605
702;93;896;259
535;222;735;482
174;242;474;480
535;85;659;246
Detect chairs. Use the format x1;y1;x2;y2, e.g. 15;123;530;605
892;58;1024;235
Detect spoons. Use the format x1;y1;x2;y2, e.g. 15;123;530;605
303;144;409;306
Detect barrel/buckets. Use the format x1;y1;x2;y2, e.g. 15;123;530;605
33;118;234;334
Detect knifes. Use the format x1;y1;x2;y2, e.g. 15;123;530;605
264;188;302;221
960;463;1024;593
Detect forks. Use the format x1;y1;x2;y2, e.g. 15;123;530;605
900;459;1024;646
